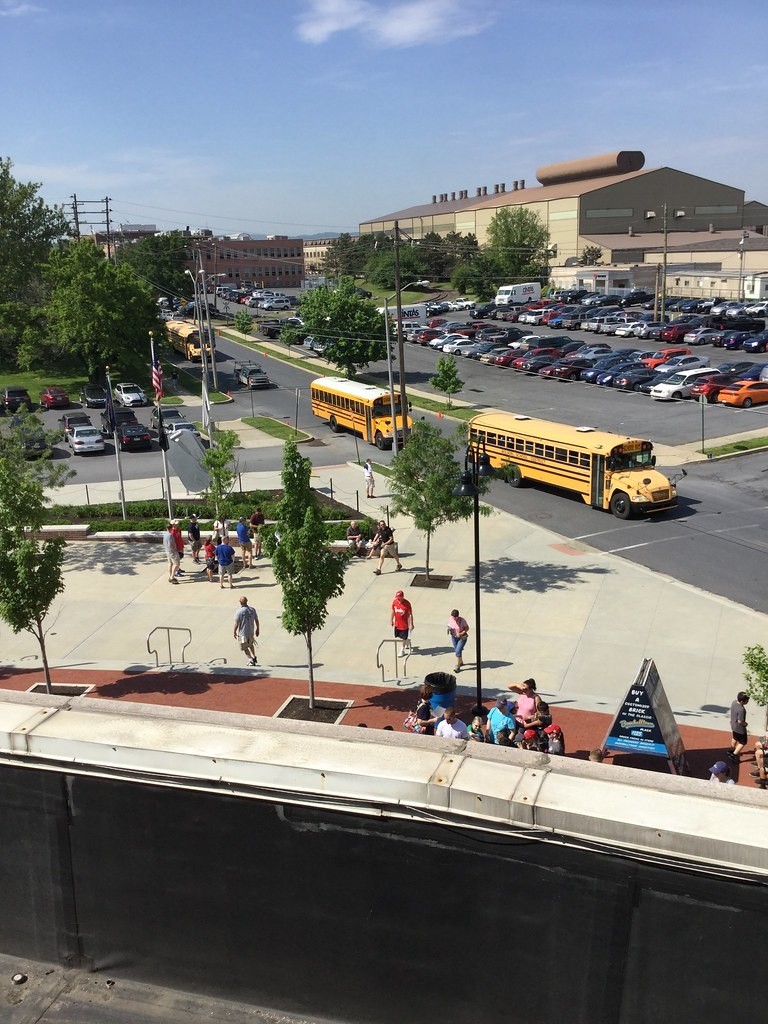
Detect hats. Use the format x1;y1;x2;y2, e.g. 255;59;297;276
709;761;727;774
544;724;561;734
239;517;246;521
170;520;179;525
496;697;507;708
395;590;404;598
524;729;537;740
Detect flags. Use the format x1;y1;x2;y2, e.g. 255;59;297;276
157;407;170;451
151;353;163;400
105;377;116;434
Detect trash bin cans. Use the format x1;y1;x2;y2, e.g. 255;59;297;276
425;671;457;729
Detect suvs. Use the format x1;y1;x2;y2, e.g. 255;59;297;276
0;386;31;412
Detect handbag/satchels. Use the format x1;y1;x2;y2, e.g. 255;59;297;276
212;530;219;542
403;703;427;734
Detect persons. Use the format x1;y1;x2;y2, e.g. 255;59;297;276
390;591;414;657
347;522;382;559
171;367;179;392
710;692;768;785
364;458;377;498
372;520;402;575
163;508;267;588
631;456;640;466
447;609;471;672
357;678;602;764
233;597;260;666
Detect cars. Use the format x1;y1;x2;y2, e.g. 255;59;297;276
215;280;291;311
79;383;148;408
469;282;768;354
354;287;477;345
57;412;105;455
257;317;333;353
100;406;152;451
150;406;202;442
9;414;53;457
39;387;70;409
443;328;768;408
156;296;219;321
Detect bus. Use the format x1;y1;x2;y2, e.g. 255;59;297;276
310;376;414;450
467;413;687;520
165;321;216;363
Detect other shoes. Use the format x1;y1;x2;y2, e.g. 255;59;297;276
373;568;381;575
255;553;263;560
175;569;185;576
247;656;257;666
367;556;371;559
727;750;740;763
398;644;409;657
454;661;463;673
395;564;402;571
193;558;202;565
749;770;760;777
168;578;180;584
367;495;376;498
755;779;768;785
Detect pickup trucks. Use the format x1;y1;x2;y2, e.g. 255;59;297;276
234;360;270;390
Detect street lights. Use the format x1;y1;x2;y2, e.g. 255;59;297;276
461;436;493;717
384;279;430;455
184;269;230;460
